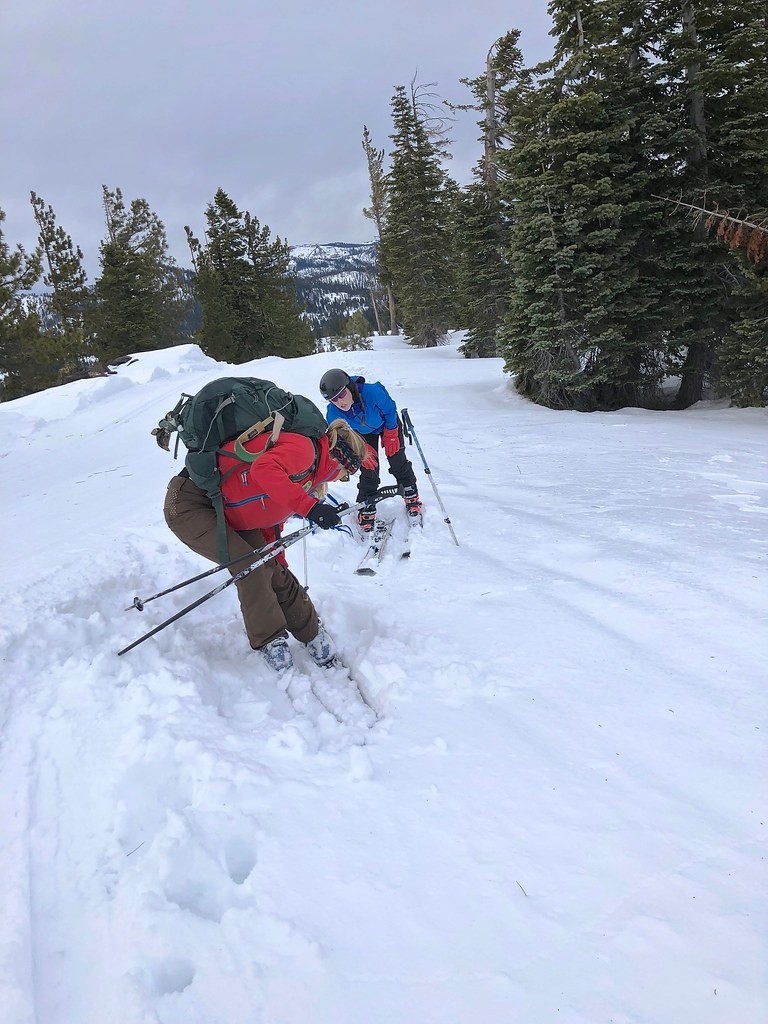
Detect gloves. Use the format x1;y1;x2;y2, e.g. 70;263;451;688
381;426;400;457
360;444;379;471
307;503;342;530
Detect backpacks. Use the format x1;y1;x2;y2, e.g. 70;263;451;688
178;376;328;499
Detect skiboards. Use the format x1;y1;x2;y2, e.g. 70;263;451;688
352;510;422;577
275;642;377;748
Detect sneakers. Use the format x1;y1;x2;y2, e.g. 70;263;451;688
405;497;423;525
306;626;338;671
357;505;377;538
260;637;293;674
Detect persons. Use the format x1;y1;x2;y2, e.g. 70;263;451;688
320;368;423;532
163;419;366;672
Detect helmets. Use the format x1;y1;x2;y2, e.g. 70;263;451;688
320;369;350;400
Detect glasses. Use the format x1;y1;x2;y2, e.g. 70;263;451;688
332;390;347;402
340;472;350;482
